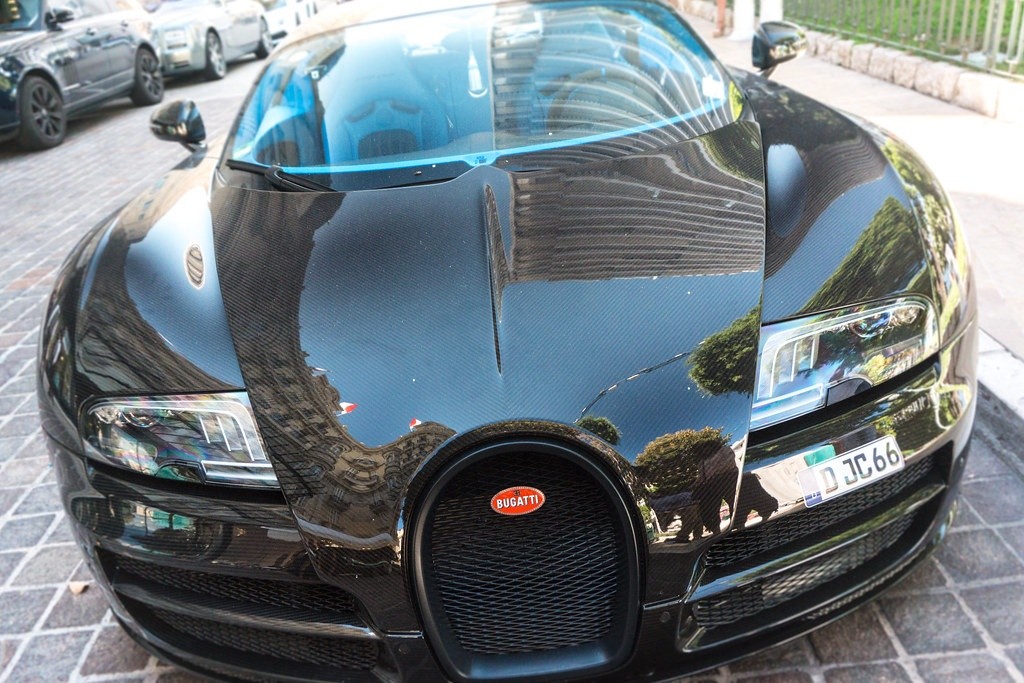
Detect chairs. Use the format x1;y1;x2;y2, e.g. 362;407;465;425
309;40;455;165
511;16;648;137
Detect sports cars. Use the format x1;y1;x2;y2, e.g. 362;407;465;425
34;0;986;683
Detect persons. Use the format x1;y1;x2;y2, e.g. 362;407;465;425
661;438;778;542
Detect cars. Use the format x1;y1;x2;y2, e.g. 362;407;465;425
0;0;336;148
